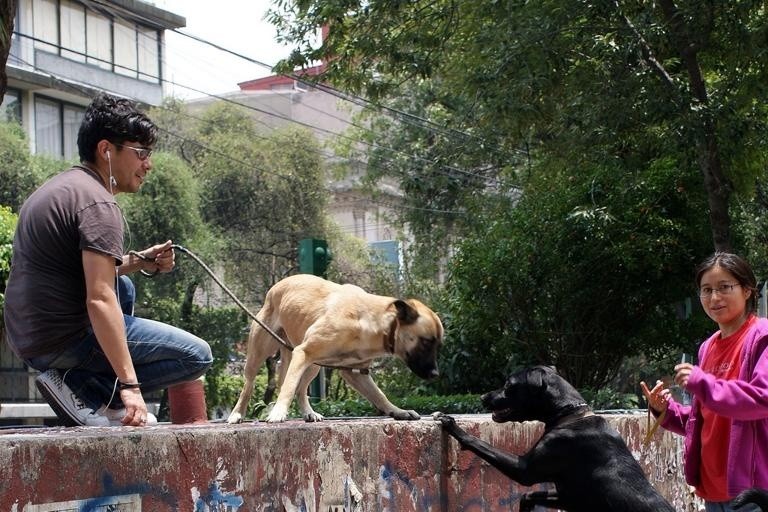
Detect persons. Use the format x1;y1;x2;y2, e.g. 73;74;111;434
639;250;768;512
1;90;218;429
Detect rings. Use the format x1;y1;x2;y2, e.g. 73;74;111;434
141;416;146;423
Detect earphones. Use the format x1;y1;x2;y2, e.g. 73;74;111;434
107;151;111;158
113;178;117;187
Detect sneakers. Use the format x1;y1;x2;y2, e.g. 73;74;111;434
34;369;158;426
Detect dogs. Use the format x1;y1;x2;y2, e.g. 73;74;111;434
430;363;678;512
226;273;445;425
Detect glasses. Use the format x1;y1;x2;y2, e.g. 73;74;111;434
697;282;741;297
117;142;153;160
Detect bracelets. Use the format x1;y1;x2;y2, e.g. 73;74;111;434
118;381;145;392
